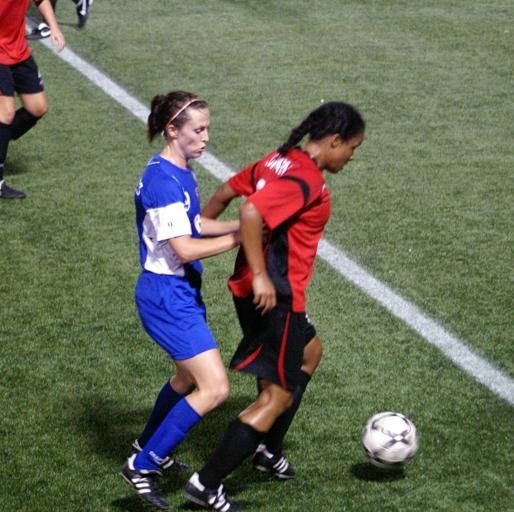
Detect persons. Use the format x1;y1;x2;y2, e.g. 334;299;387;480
0;0;66;199
24;0;93;39
119;91;266;510
181;102;365;511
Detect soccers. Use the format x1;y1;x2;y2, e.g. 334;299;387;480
361;411;418;468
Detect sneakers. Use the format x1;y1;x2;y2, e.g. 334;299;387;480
0;179;26;199
251;443;296;479
24;22;51;41
182;471;239;512
119;438;189;510
75;0;93;29
